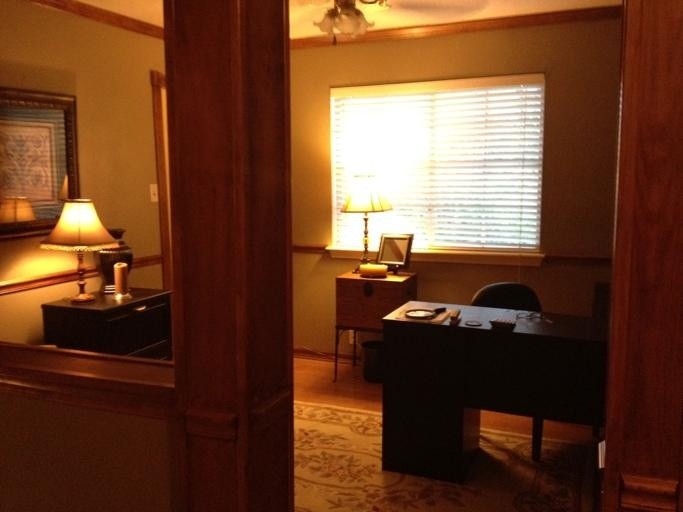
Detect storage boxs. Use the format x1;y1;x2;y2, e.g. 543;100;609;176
336;270;416;329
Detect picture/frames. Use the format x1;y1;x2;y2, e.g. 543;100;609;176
0;84;81;240
376;232;414;268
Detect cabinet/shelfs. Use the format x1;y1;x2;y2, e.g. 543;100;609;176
41;286;172;360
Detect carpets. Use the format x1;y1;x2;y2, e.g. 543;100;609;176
294;400;599;512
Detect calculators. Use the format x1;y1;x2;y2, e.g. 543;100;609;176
489;318;516;327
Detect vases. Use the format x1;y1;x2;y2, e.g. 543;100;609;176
100;228;132;295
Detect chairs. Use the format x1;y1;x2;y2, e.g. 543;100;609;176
471;281;601;462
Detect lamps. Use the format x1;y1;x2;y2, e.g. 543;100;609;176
57;174;68;200
339;174;393;272
313;0;375;42
40;197;120;303
0;197;36;222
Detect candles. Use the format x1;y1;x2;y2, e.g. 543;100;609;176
113;262;130;295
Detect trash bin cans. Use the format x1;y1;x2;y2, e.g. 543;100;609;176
363;341;383;384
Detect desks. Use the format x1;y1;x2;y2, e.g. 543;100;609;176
380;301;606;484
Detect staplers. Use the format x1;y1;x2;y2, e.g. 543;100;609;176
449;309;462;326
